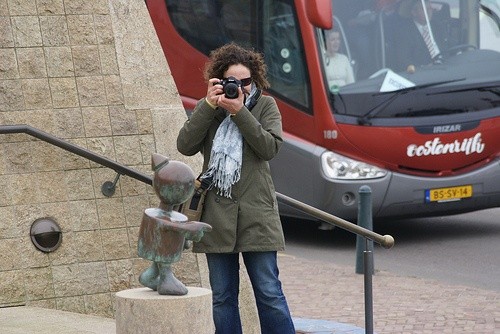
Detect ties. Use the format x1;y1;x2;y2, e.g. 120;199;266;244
423;28;435;60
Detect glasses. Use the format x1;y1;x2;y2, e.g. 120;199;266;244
240;76;253;87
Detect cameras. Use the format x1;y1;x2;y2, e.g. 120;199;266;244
215;77;242;99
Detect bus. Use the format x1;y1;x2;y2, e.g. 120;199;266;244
144;0;500;232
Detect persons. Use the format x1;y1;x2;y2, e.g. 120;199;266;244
398;0;459;71
318;27;356;89
176;43;298;333
135;151;213;295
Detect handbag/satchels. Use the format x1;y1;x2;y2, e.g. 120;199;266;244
176;174;212;222
